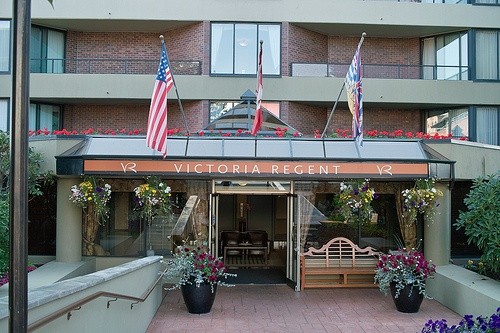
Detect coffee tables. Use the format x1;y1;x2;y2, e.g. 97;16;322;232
224;244;268;269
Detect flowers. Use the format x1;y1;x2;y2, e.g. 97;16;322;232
127;176;179;225
69;178;112;227
157;232;238;294
334;178;376;225
401;179;443;227
370;238;437;301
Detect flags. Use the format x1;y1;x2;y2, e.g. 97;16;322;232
252;42;264;137
145;40;177;159
344;41;365;160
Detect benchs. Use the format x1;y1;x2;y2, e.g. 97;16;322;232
300;237;381;290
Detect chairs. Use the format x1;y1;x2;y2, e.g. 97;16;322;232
245;230;270;257
220;230;244;257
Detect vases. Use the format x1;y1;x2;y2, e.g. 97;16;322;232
182;276;217;315
390;280;426;313
